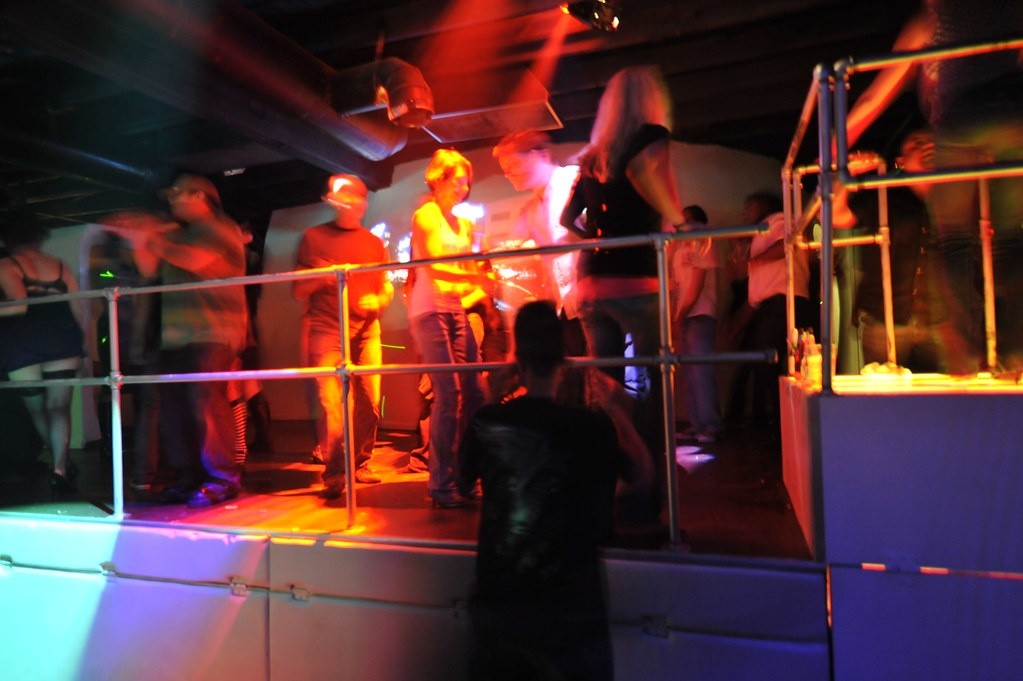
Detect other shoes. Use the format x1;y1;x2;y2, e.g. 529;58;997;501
693;423;726;441
186;478;241;506
396;463;416;473
469;487;485;501
39;468;72;501
354;464;382;484
673;423;701;441
320;474;344;500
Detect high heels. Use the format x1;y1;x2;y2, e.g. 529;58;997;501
431;489;468;509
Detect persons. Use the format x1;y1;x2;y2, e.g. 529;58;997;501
558;67;705;505
721;131;983;433
670;204;723;443
396;128;646;508
290;173;394;490
0;210;87;493
454;301;654;681
99;177;254;507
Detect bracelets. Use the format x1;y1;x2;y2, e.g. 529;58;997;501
672;215;687;229
